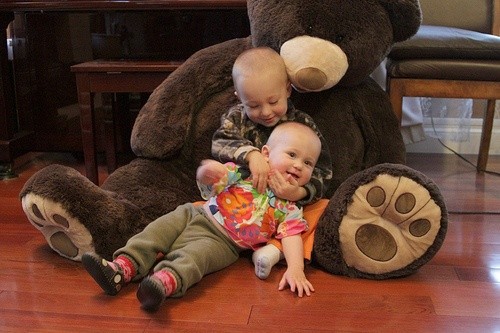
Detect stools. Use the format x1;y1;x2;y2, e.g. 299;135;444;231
69;59;186;187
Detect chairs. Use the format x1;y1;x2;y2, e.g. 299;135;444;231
386;0;500;173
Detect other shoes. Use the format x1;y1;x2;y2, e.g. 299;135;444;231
136;278;166;310
82;252;126;295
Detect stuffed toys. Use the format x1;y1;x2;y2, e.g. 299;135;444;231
19;0;449;281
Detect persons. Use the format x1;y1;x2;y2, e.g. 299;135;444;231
211;46;332;279
82;122;322;316
